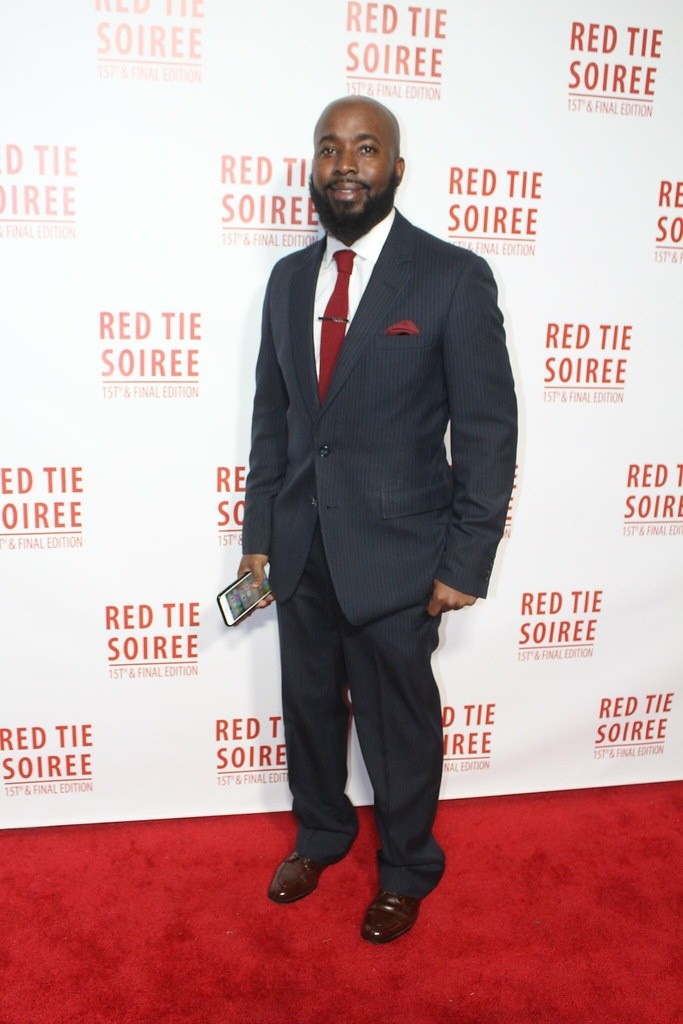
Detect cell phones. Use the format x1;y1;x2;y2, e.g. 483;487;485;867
218;561;272;626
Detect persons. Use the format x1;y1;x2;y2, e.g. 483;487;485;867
234;96;517;945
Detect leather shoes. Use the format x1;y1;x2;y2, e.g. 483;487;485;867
362;876;418;941
270;845;323;902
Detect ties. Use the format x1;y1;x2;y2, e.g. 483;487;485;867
319;246;355;405
